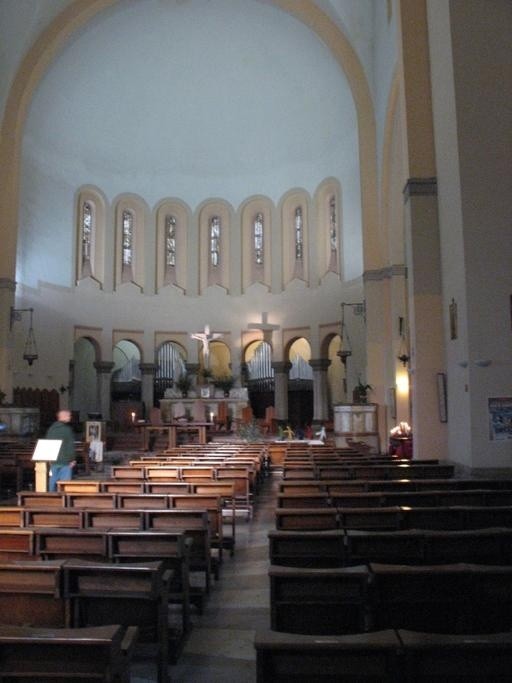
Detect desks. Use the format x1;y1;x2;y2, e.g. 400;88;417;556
136;422;214;451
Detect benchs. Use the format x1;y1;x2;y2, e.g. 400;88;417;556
251;441;512;681
1;441;271;682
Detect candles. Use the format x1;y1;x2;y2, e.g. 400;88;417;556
131;412;136;422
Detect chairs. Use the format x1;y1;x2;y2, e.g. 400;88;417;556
149;404;277;434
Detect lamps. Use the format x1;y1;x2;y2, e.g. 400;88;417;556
10;306;38;367
337;300;366;365
398;315;410;368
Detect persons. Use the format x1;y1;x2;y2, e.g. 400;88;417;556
43;407;80;492
191;334;221;368
392;422;412;456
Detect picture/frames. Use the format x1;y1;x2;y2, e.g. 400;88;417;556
388;388;397;419
436;373;447;422
449;303;458;340
